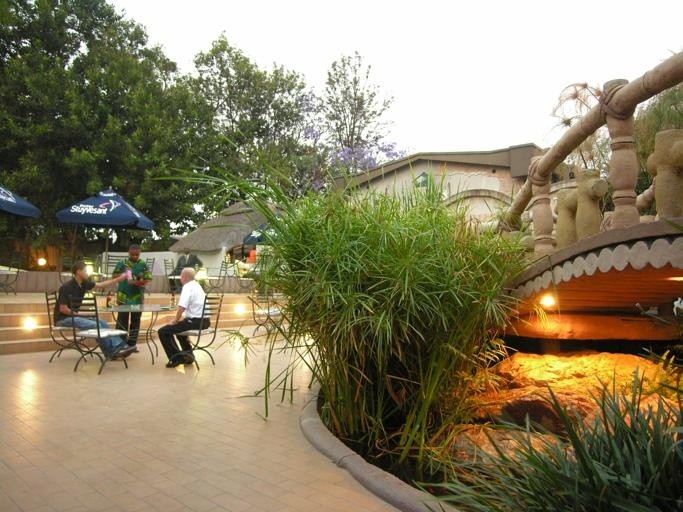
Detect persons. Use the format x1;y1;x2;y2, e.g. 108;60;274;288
170;244;204;294
52;260;136;360
157;266;211;369
110;243;152;353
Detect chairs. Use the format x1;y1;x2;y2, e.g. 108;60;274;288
44;254;228;375
0;262;19;296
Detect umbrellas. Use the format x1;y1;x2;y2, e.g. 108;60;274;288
244;214;288;248
53;184;155;276
0;185;43;221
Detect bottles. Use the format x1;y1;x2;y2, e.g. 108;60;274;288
107;290;112;308
170;289;176;308
126;266;133;281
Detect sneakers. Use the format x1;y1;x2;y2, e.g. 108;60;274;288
166;357;184;367
106;346;139;360
